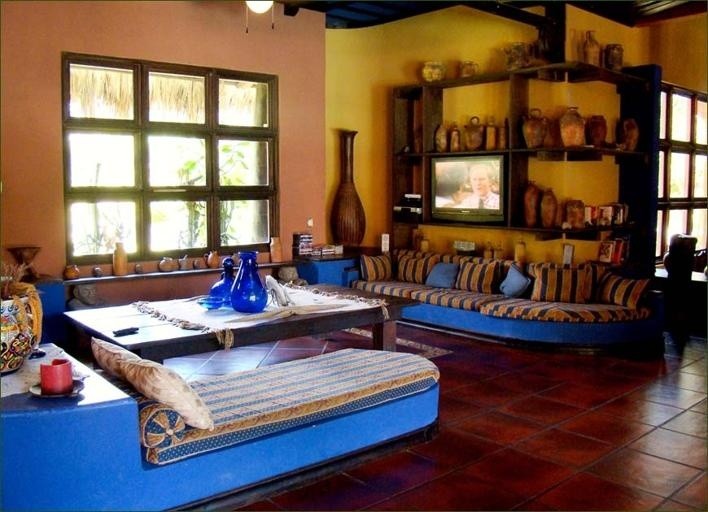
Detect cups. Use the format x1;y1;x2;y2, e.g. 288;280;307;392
41;359;73;393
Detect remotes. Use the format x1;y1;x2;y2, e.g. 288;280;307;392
113;327;139;335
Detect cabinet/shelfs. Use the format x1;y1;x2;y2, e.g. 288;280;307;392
392;61;662;276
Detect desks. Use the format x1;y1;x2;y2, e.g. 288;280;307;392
64;283;420;364
653;267;707;339
292;253;381;289
36;279;66;343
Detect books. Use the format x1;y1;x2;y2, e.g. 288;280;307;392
598;235;630;265
597;201;629;225
292;232;344;256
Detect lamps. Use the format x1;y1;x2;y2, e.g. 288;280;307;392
243;1;274;35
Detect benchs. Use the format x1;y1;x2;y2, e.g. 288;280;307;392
0;342;441;512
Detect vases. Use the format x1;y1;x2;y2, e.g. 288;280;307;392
421;60;447;84
269;236;283;263
6;247;42;280
229;250;268;313
0;282;43;376
112;242;128;276
540;186;557;228
331;129;366;247
560;106;586;147
524;179;541;226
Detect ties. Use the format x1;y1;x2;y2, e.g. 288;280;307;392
478;199;482;209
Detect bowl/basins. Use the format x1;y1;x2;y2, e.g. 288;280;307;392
198;297;223;309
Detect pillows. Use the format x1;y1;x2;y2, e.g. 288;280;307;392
360;254;650;309
91;336;214;431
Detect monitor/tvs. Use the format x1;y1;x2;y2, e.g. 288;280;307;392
431;156;506;223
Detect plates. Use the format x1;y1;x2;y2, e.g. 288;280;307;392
29;379;83;398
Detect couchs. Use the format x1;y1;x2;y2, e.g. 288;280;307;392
344;246;664;361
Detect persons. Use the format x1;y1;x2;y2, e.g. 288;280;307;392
434;161;499;211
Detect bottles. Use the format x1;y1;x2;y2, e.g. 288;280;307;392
450;125;460;152
485;121;496;150
